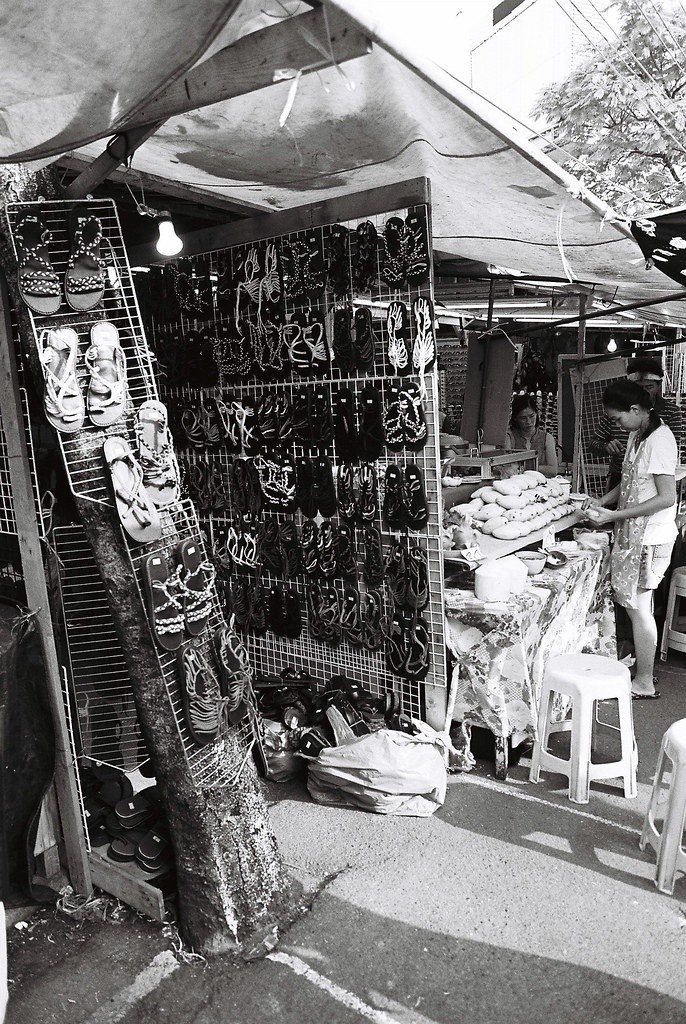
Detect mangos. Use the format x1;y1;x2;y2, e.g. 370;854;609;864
448;470;577;540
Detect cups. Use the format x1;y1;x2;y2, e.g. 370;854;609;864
554;475;570;495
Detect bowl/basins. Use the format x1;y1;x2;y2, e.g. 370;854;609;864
545;551;568;569
569;496;586;509
515;551;547;575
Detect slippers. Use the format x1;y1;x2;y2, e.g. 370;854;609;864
12;193;427;877
614;687;662;700
631;671;659;686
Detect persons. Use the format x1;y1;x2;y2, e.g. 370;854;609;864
581;379;680;701
505;394;559;478
590;357;682;530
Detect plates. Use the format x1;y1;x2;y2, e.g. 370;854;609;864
462;480;482;483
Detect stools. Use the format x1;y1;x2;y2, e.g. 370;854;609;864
661;566;686;661
639;718;686;895
529;653;640;805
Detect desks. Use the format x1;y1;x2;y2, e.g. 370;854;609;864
442;528;618;779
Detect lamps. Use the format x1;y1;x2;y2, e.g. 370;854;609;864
607;332;617;353
155;211;184;256
